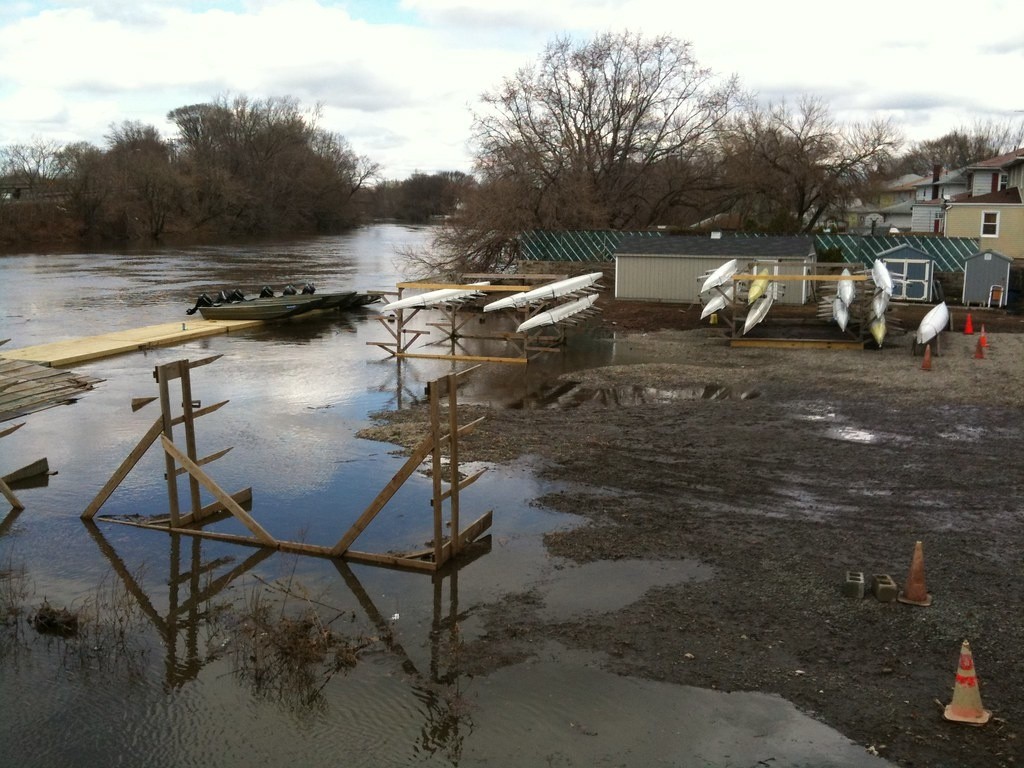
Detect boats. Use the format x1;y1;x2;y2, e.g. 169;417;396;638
867;259;893;346
380;282;491;315
832;267;856;331
254;286;358;310
701;257;739;297
516;293;601;339
747;268;770;306
699;281;742;320
743;292;774;336
483;269;603;314
184;286;325;320
916;301;951;345
259;284;384;311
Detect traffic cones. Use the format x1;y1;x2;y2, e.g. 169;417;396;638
963;314;974;335
920;344;935;370
896;540;933;607
972;338;986;360
976;323;990;347
942;638;993;727
